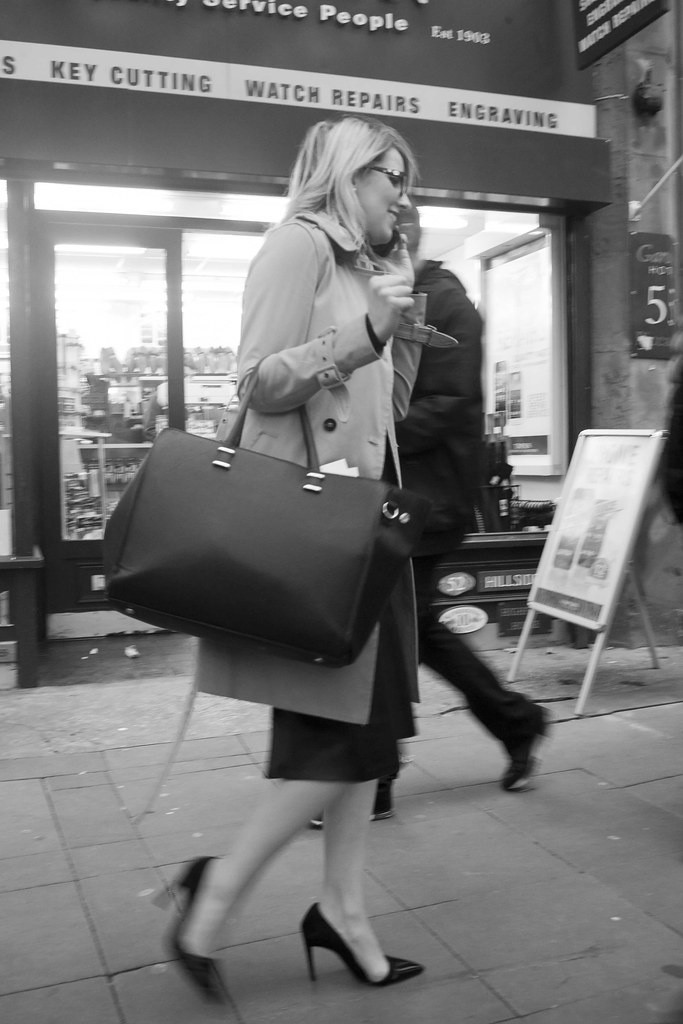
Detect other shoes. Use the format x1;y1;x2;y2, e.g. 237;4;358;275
502;705;545;789
309;784;393;826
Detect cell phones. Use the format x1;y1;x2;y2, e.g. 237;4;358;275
370;230;400;257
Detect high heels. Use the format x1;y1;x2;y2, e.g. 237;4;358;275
302;902;425;987
153;856;222;1003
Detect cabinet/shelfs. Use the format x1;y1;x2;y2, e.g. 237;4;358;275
58;436;152;541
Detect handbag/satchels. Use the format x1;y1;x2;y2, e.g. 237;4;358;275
101;357;433;669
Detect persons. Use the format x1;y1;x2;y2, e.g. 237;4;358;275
157;116;424;1003
306;203;552;829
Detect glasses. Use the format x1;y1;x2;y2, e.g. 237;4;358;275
366;166;408;196
